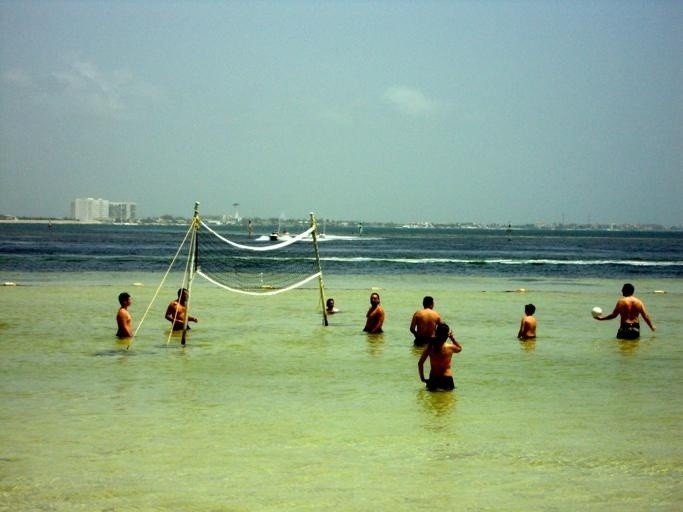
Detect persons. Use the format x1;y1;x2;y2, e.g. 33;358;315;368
408;295;442;346
593;282;656;340
361;292;385;334
114;291;133;338
163;287;198;332
324;297;338;312
517;303;537;339
416;323;463;392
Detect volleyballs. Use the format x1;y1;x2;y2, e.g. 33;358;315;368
592;307;602;317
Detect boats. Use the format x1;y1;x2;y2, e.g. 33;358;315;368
269;230;289;240
